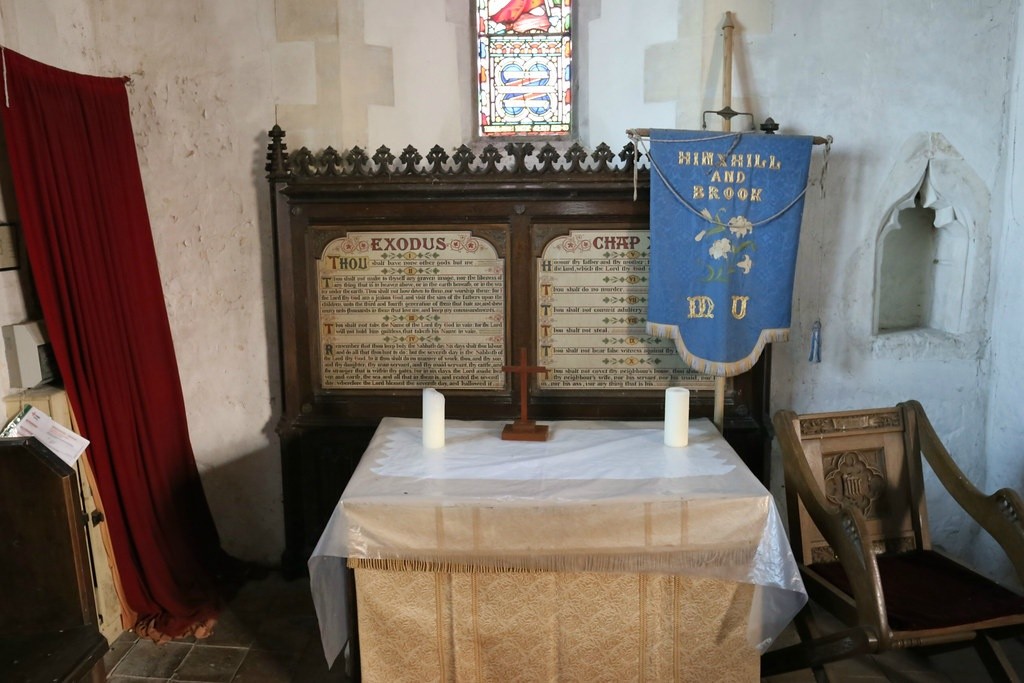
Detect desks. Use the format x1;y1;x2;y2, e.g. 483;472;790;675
309;415;807;683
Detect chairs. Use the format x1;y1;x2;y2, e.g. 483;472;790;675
759;400;1024;683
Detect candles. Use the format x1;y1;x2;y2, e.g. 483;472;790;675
422;387;445;447
664;387;690;447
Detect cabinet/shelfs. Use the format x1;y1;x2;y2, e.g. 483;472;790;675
0;390;123;683
